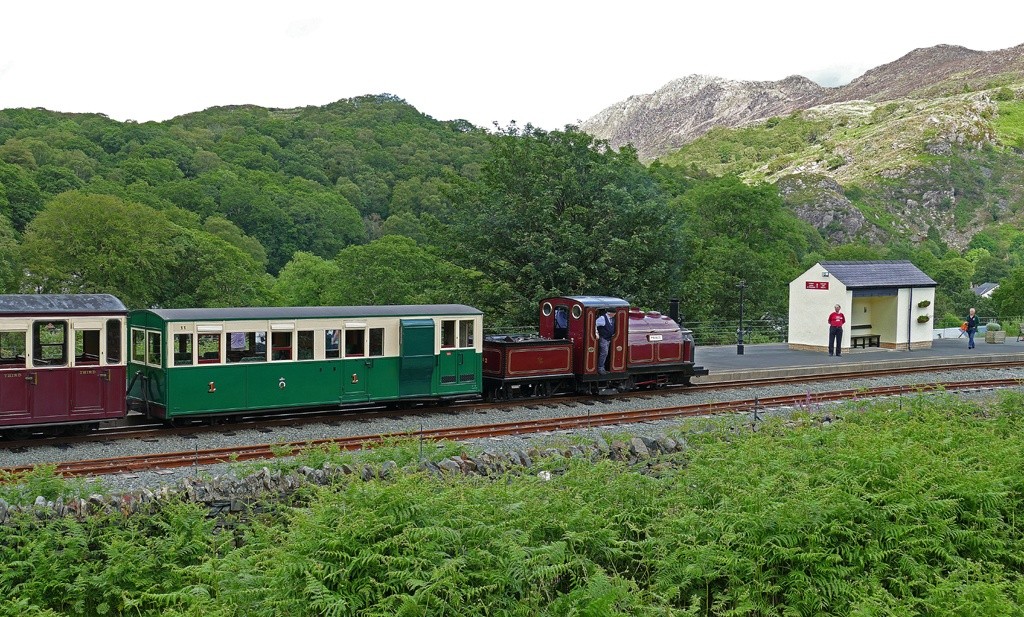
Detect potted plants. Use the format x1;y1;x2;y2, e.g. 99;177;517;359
984;323;1006;344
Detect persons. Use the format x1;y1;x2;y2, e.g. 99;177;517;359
594;308;617;374
828;303;846;356
966;308;979;349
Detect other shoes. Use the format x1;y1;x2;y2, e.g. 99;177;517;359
597;369;607;375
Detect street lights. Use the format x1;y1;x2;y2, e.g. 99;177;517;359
735;280;749;355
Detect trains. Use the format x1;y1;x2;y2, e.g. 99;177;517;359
0;293;707;434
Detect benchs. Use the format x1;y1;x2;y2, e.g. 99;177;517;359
851;334;881;349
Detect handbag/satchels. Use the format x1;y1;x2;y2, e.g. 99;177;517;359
961;323;968;331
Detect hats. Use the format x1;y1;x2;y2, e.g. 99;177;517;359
608;308;616;313
